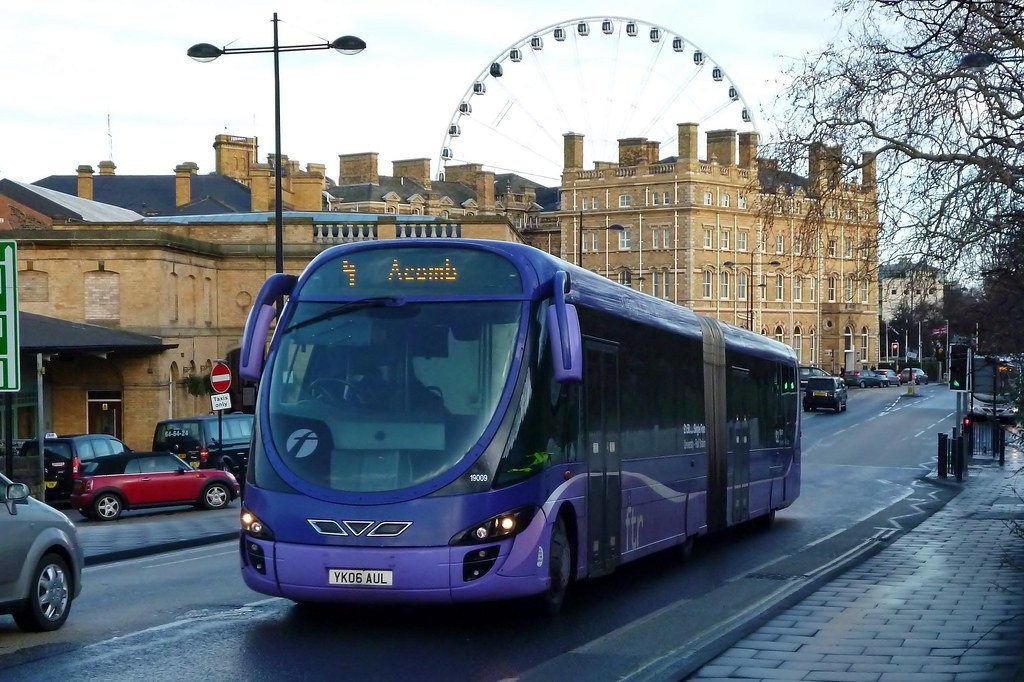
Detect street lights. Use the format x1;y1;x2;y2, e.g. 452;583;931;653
184;13;368;326
746;273;767;330
723;252;782;333
578;211;625;267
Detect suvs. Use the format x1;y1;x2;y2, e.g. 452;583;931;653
16;432;137;509
151;411;255;496
802;375;849;413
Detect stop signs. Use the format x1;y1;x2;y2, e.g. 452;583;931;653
209;363;232;394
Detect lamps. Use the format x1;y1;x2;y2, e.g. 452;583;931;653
98;352;107;359
44;352;58;362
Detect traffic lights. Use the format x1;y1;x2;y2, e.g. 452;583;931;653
948;344;969;392
892;342;899;359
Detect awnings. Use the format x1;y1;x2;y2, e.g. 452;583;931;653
19;310;181;351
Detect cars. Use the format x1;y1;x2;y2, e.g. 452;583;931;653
873;369;903;387
841;370;885;388
799;366;832;387
898;367;928;386
68;450;241;522
0;472;84;634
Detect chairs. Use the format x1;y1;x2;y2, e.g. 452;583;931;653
352;360;393;396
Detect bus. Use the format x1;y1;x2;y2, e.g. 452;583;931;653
238;238;803;618
